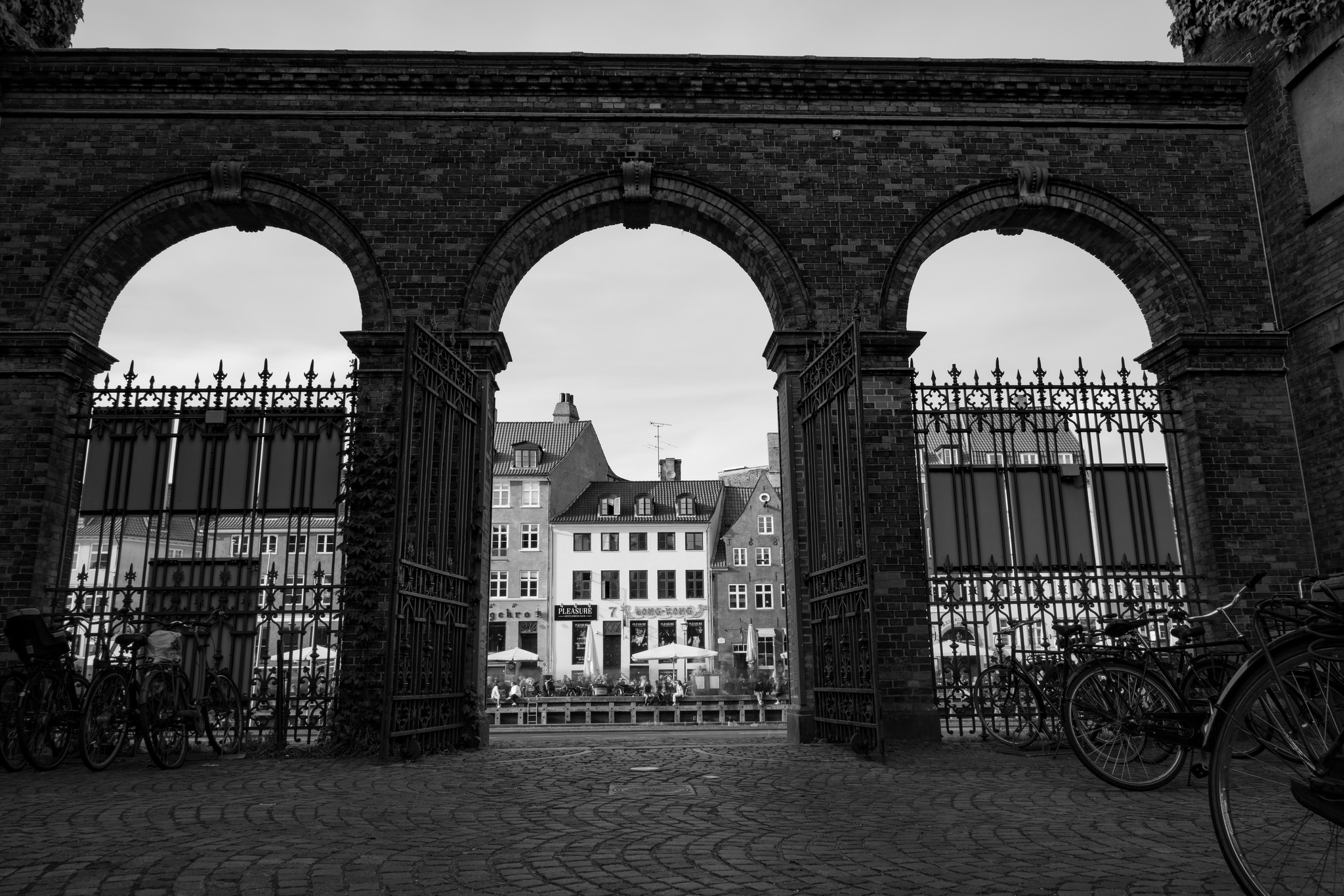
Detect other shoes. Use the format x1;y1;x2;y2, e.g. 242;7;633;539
758;701;764;705
666;702;670;706
497;705;500;708
647;703;650;706
672;703;677;706
512;702;517;706
775;701;780;705
659;703;663;706
644;704;647;706
506;697;511;701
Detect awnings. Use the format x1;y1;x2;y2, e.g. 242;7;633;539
782;628;787;637
756;628;776;637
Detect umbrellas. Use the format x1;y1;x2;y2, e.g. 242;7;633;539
270;645;337;682
1092;638;1146;652
1041;622;1049;651
746;624;757;678
488;647;544;683
583;624;599;682
929;640;994;656
1169;627;1179;646
631;641;718;688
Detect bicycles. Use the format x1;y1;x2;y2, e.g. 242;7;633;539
973;568;1344;896
0;603;245;772
526;676;693;698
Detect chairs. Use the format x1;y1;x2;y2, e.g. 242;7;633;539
487;683;527;697
634;685;644;697
682;683;697;696
558;684;592;697
742;682;787;695
655;680;659;693
607;682;614;696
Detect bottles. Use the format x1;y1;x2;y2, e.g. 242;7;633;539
693;665;703;675
712;669;719;675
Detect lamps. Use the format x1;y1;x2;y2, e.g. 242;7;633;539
588;603;591;607
574;604;577;607
535;608;541;617
763;501;768;507
512;603;517;608
489;603;495;608
560;604;563;607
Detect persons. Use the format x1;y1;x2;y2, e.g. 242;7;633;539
487;673;527;698
283;675;334;696
688;625;704;648
722;671;788;708
631;627;647;654
577;627;586;664
617;675;696;706
491;681;505;708
659;627;675;646
506;681;522;705
935;670;978;693
555;674;614;697
